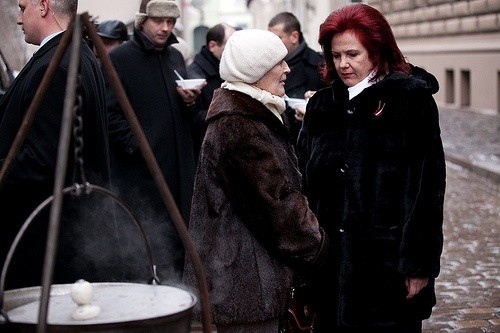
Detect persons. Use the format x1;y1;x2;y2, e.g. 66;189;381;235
293;4;447;332
100;0;205;290
179;28;330;332
81;7;338;151
1;0;126;332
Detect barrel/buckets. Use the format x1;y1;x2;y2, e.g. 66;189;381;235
0;187;199;333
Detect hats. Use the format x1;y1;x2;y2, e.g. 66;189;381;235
219;28;288;84
134;0;181;30
95;20;129;42
